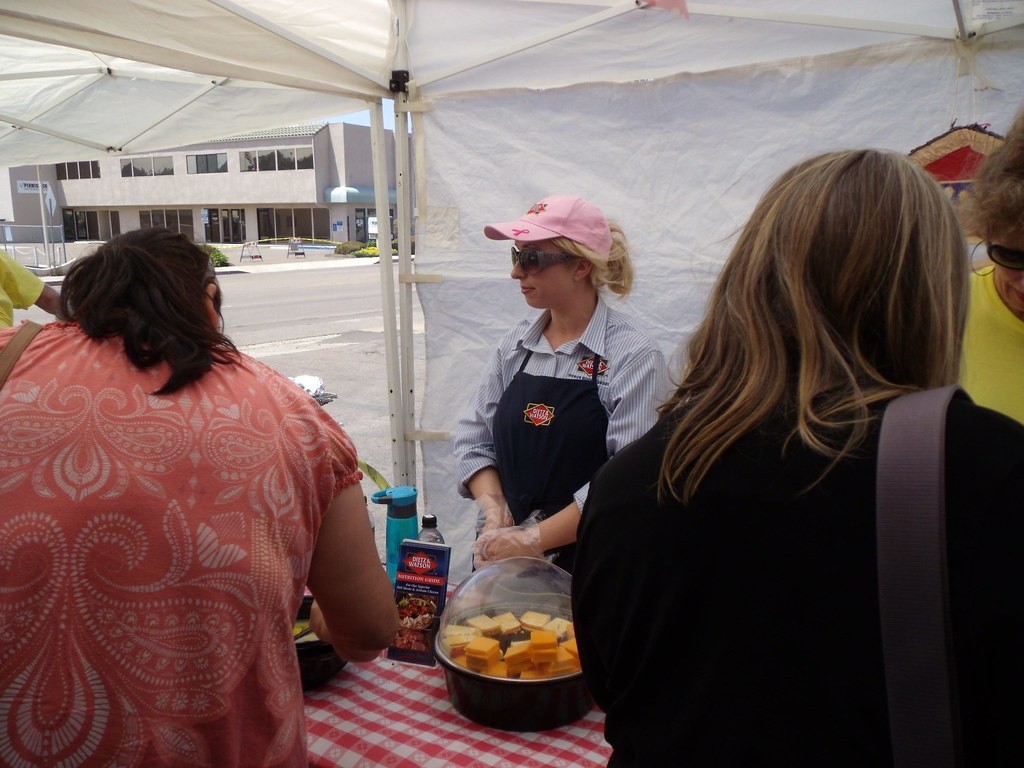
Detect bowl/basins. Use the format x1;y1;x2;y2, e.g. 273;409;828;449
431;555;595;733
293;594;348;693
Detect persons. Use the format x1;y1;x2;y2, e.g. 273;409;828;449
0;247;60;336
451;194;673;582
569;146;1023;768
0;227;402;767
955;107;1024;424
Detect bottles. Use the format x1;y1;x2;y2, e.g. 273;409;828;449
418;513;445;545
371;485;420;590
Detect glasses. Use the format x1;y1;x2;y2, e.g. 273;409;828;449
987;242;1024;270
511;247;583;275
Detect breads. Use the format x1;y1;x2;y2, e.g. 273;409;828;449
441;611;583;680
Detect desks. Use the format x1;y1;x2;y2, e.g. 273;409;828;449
300;578;617;768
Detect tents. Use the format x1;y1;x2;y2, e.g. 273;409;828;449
0;1;1024;584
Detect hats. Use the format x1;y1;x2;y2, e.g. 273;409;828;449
484;194;612;261
